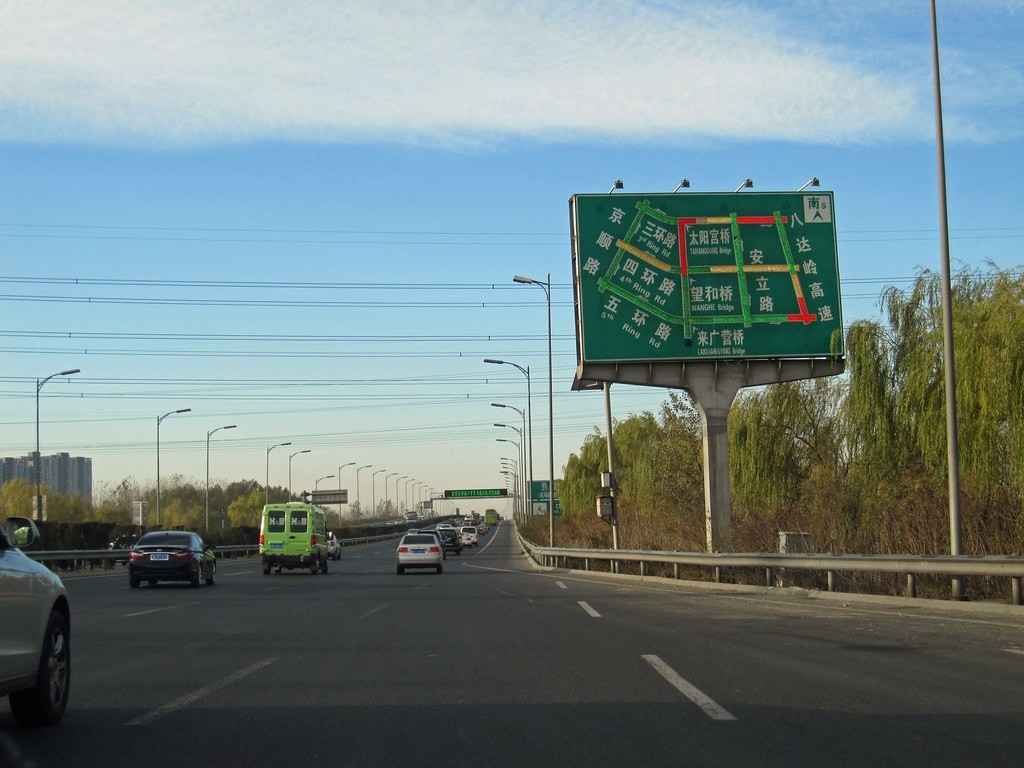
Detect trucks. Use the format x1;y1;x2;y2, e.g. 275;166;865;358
259;502;329;575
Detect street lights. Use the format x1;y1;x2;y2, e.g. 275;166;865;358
289;450;311;503
396;476;407;521
385;472;398;522
514;274;553;567
373;469;386;523
157;408;191;530
483;360;533;517
316;475;335;508
205;425;237;531
405;479;434;510
338;462;356;527
266;442;292;505
493;424;524;525
357;465;372;525
491;403;527;525
37;369;80;524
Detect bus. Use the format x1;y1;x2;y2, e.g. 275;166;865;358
483;509;497;525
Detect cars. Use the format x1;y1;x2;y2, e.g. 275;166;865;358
394;512;490;575
0;516;72;727
128;531;216;587
327;531;341;559
